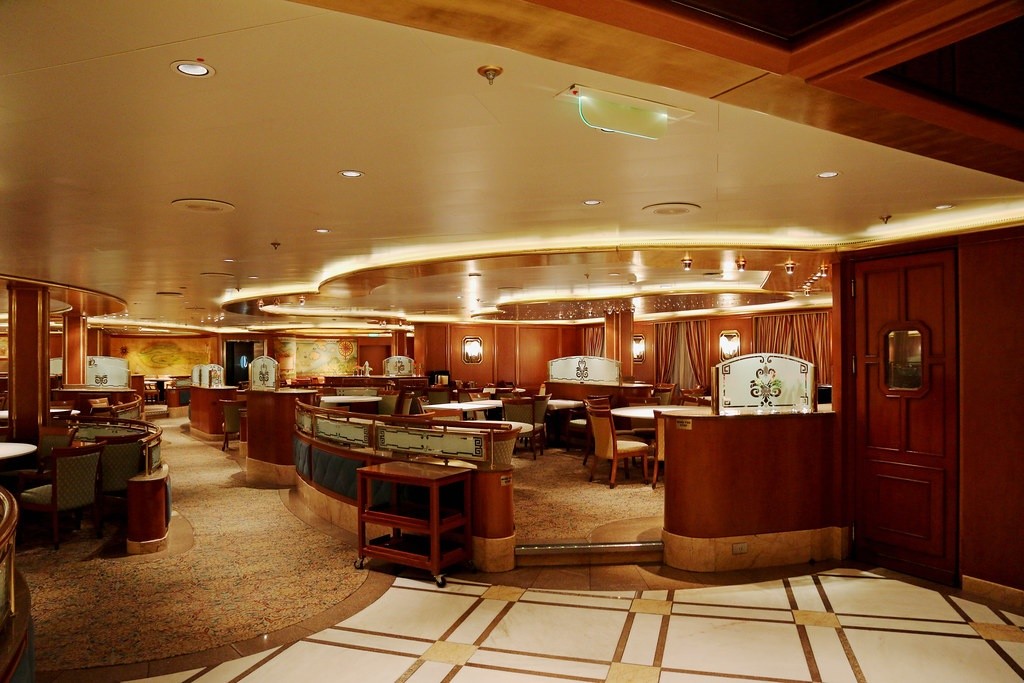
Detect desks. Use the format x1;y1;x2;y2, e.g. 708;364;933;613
145;378;177;403
423;401;496;421
0;407;81;419
611;405;706;420
472;399;503;420
482;420;532;435
547;399;585;412
319;395;383;412
0;441;37;463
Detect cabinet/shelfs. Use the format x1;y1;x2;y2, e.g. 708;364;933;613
355;462;479;589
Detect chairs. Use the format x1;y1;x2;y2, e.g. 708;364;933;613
564;379;712;489
12;426;80;487
145;386;160;404
279;376;552;460
17;439;108;550
95;430;150;524
219;399;247;452
